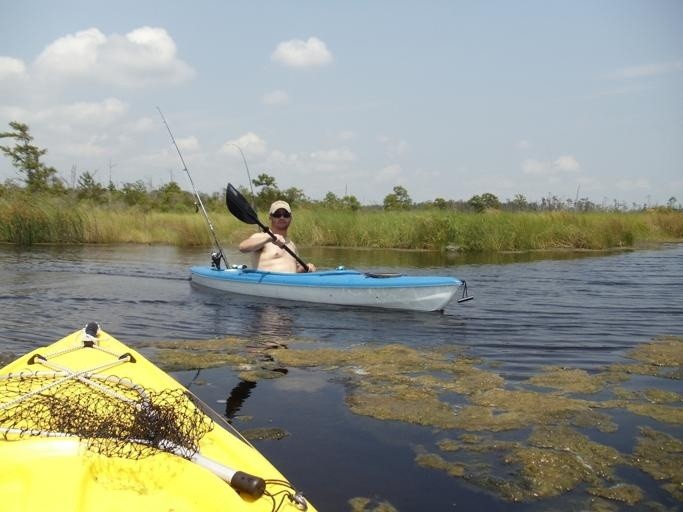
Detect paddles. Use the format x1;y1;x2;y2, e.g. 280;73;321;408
226;183;310;273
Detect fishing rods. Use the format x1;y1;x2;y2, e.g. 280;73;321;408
155;104;230;269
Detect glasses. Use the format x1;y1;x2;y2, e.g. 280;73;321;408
272;213;289;217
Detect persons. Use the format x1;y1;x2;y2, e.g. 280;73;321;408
237;195;316;275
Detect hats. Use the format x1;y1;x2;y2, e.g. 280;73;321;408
269;201;291;215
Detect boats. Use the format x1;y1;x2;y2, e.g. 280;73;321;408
0;316;319;512
185;259;464;315
